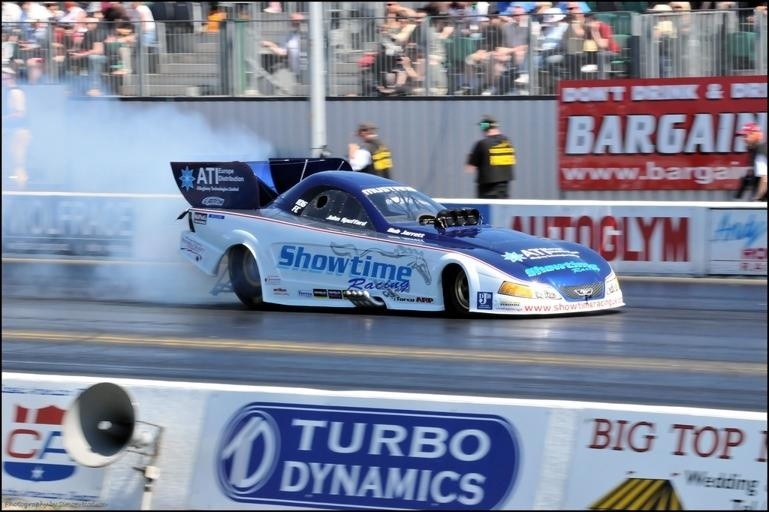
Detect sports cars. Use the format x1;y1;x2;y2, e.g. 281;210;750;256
169;157;627;316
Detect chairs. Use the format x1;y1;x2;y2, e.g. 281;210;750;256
440;2;757;95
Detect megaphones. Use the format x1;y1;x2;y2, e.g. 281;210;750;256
61;381;164;470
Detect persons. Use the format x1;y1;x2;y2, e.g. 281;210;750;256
259;0;767;96
735;123;767;202
466;116;517;198
1;1;156;98
205;2;252;97
348;124;393;179
2;66;35;192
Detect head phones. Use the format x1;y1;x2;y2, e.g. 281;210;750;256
480;122;500;131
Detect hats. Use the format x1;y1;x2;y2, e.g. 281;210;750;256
355;122;379;133
734;122;762;136
